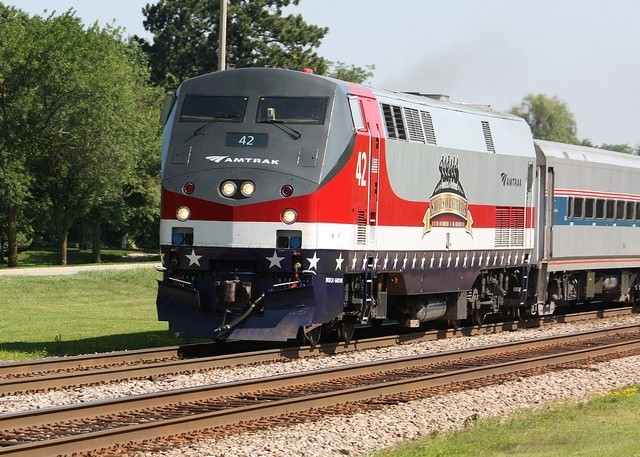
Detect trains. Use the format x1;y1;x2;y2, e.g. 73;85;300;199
157;67;640;347
